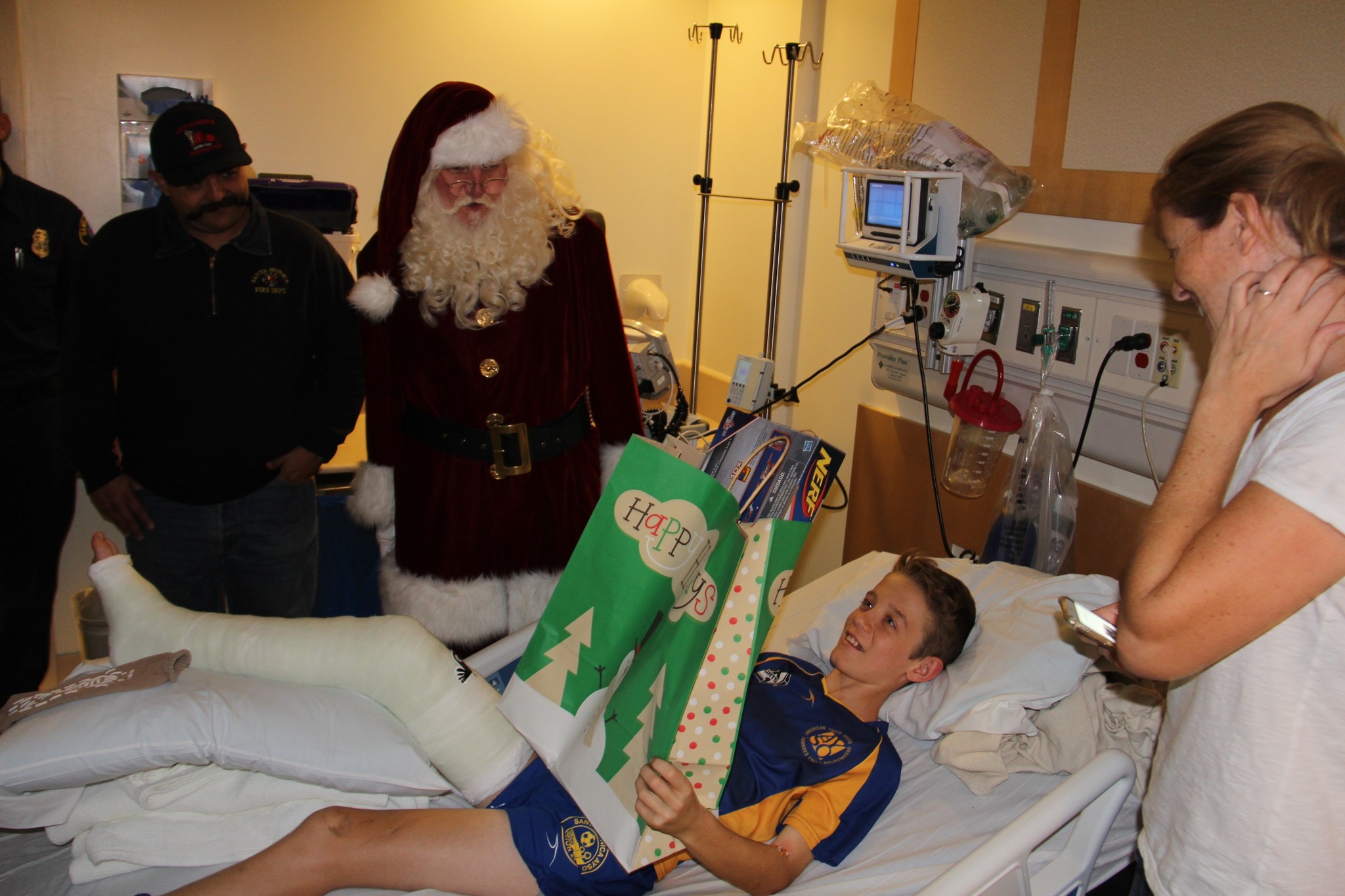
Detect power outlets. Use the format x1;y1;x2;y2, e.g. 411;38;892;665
973;290;1189;393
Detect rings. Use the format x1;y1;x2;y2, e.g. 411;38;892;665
1255;286;1278;298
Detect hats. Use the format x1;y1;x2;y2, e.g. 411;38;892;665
149;101;253;188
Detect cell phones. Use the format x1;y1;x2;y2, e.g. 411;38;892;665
1057;594;1119;654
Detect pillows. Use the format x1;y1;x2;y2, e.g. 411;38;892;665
3;652;453;797
784;543;1130;746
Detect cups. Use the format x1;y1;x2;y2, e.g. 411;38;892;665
943;348;1023;499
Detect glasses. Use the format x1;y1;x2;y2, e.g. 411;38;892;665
439;172;509;195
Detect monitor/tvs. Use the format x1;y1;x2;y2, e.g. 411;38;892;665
858;174;929;248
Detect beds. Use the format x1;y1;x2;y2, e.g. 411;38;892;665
0;400;1189;896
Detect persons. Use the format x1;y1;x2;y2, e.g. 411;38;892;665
1072;100;1345;896
58;99;374;619
0;89;101;717
86;528;978;896
353;81;646;652
